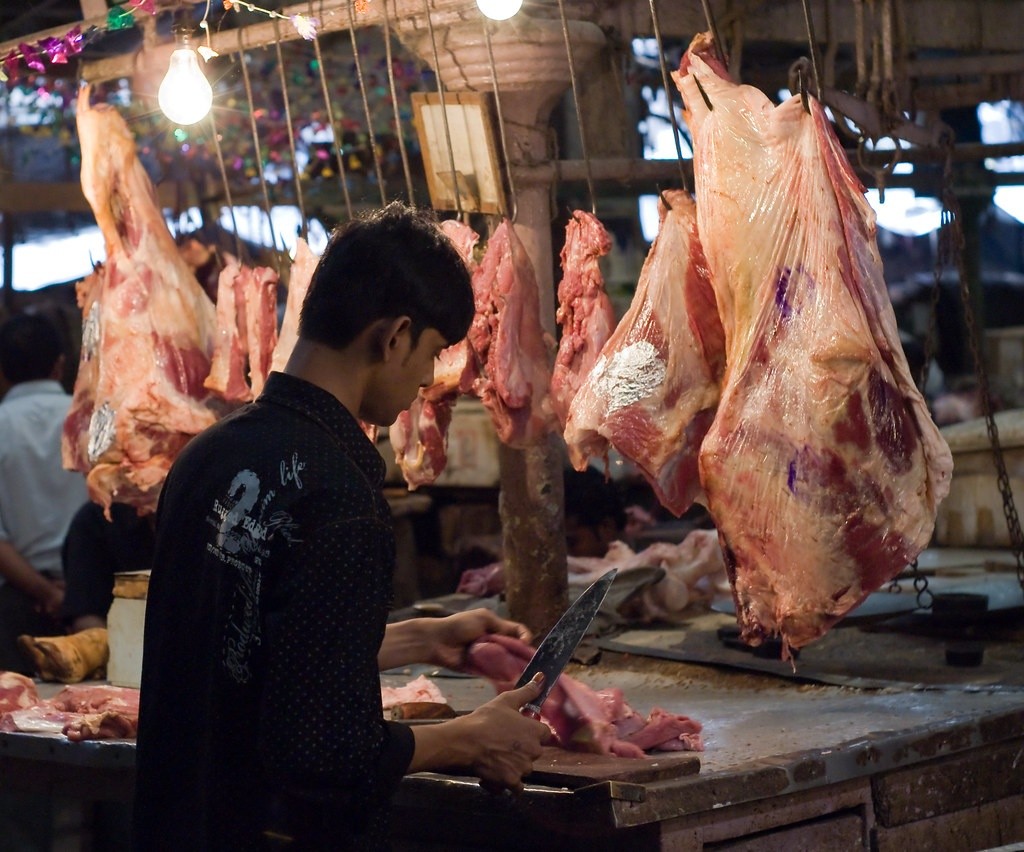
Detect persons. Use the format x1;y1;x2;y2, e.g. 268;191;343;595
564;464;652;559
185;224;226;306
133;203;552;852
0;312;90;679
58;497;157;680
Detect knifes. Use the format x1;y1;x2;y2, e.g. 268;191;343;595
513;568;618;721
